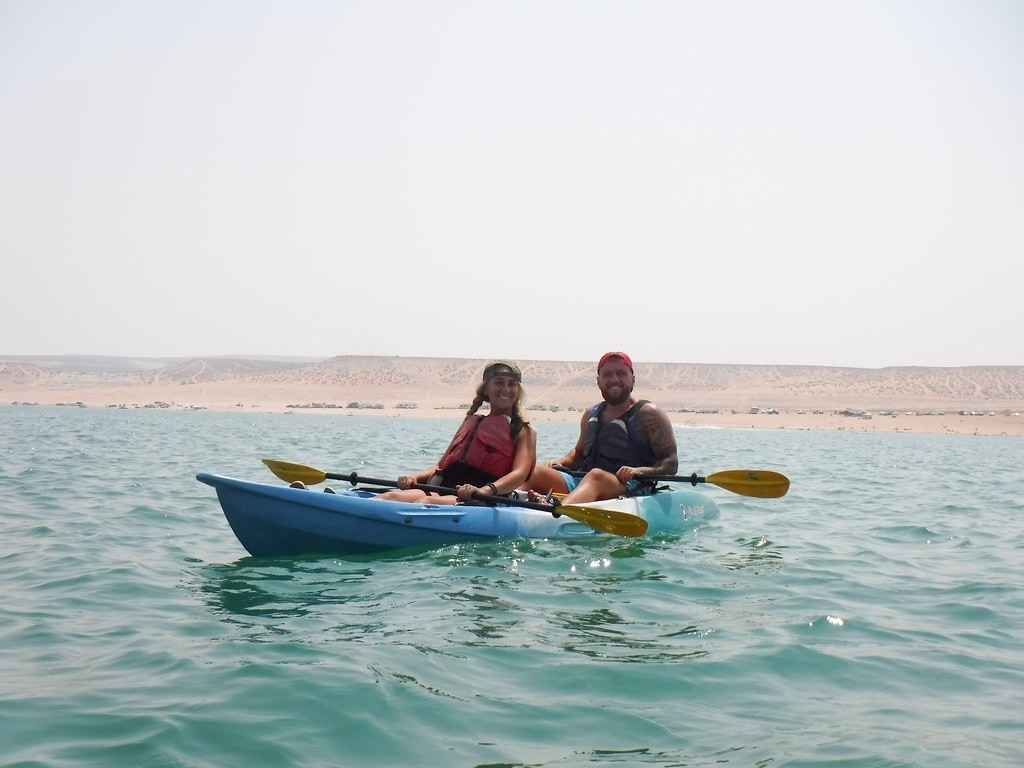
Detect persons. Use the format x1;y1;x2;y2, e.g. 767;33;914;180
289;359;537;504
516;351;678;505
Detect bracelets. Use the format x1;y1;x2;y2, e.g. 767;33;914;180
486;484;497;494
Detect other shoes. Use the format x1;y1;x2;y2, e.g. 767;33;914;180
290;481;306;490
324;486;338;495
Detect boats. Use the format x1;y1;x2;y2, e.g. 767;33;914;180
195;469;718;558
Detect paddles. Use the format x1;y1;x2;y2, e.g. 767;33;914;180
570;468;792;499
260;454;649;538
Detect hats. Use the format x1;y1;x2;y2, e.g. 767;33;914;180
598;351;634;375
482;359;521;383
